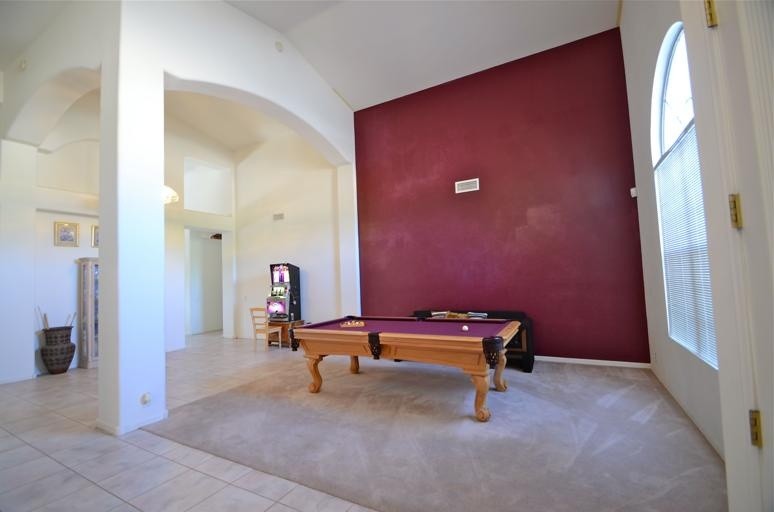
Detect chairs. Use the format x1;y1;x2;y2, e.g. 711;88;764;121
249;307;283;352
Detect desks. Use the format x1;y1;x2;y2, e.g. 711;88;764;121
289;314;521;422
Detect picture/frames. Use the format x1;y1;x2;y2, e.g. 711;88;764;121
91;224;99;248
53;220;80;247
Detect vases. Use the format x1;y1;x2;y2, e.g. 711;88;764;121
40;326;77;374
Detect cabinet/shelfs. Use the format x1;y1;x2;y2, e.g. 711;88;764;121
267;319;303;349
77;256;99;369
266;263;300;321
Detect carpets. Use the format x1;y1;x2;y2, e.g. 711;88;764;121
137;355;726;512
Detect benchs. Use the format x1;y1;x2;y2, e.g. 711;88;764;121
394;308;535;374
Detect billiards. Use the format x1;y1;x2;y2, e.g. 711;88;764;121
463;326;468;332
342;319;364;326
